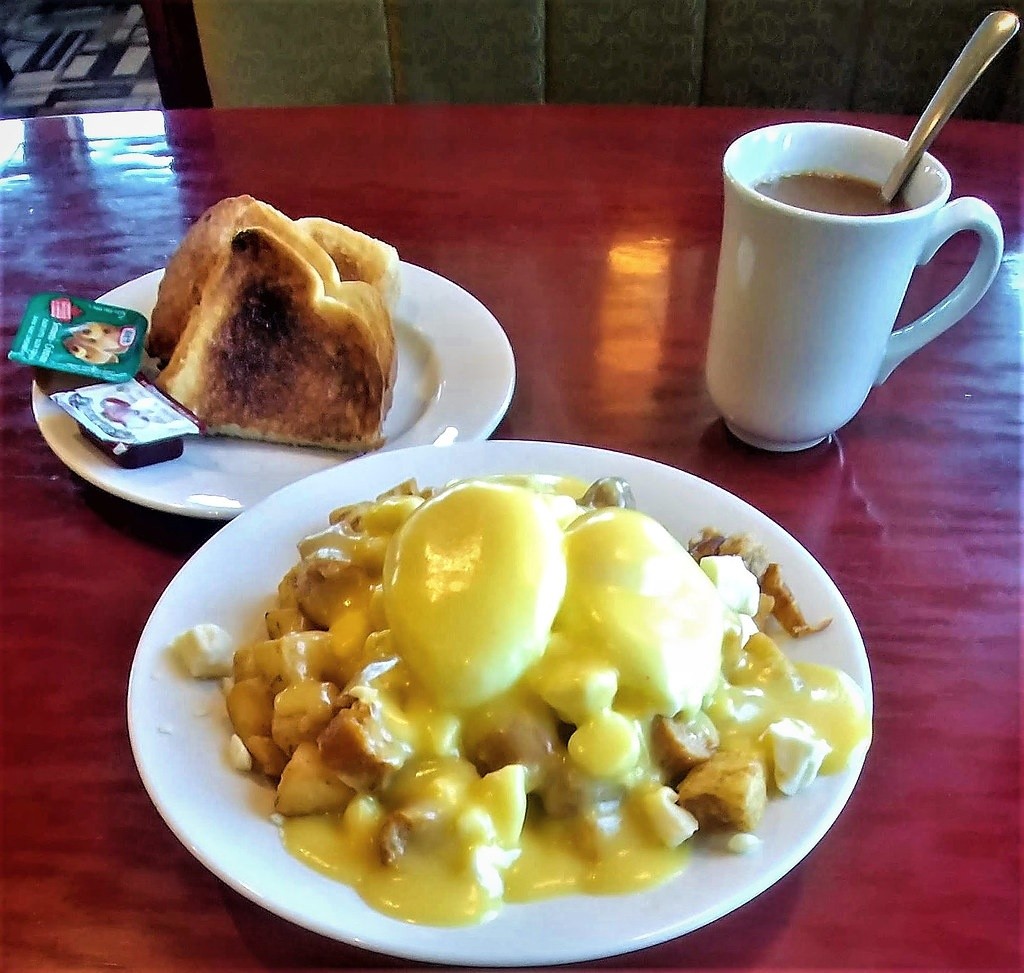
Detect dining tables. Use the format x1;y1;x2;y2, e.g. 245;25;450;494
0;106;1024;973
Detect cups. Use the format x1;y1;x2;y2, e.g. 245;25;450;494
704;121;1004;454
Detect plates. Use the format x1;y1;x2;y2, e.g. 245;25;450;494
32;261;517;519
126;438;874;968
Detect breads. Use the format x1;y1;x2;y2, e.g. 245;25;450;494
143;194;399;451
